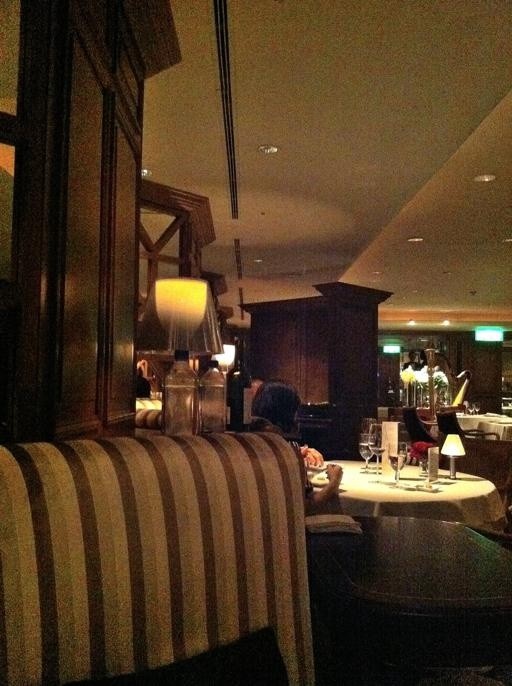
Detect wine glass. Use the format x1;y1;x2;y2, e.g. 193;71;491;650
386;441;410;488
358;418;388;484
467;401;481;416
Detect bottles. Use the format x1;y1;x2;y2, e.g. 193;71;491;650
197;361;227;436
163;349;199;438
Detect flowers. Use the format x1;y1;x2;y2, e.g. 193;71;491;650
407;439;435;471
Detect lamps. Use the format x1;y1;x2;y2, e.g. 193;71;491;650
132;277;225;437
438;430;468;477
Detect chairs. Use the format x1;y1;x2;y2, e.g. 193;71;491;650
401;405;501;445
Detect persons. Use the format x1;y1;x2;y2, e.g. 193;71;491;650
401;350;420;371
137;362;153;398
248;378;342;517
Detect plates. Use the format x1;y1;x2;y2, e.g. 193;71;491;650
400;483;440;492
